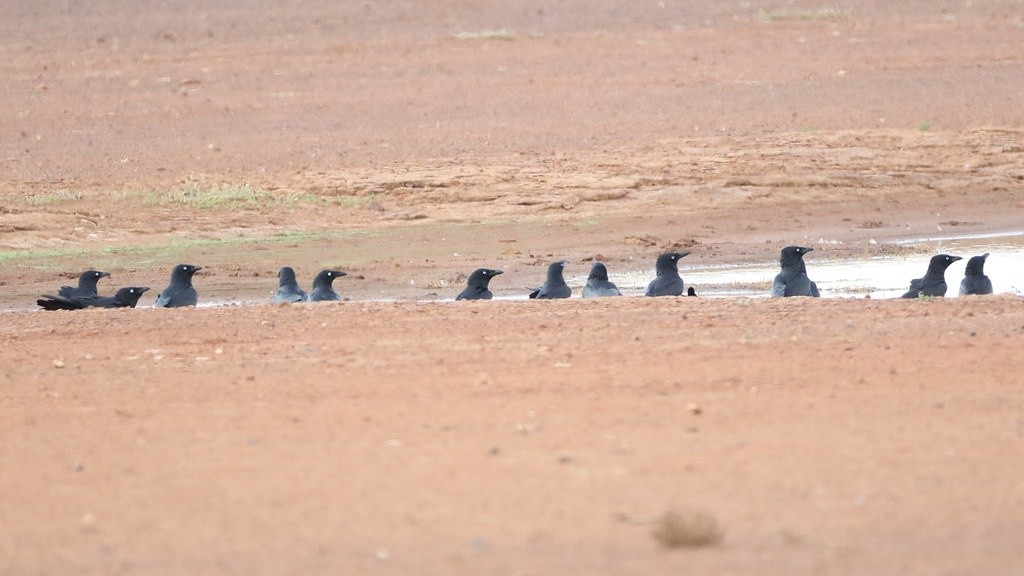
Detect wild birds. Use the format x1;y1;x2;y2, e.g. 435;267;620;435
644;251;697;297
582;261;624;297
900;253;963;299
36;270;150;310
154;264;202;307
305;269;348;302
529;258;572;301
958;252;993;296
270;266;308;304
770;245;821;298
454;268;503;300
